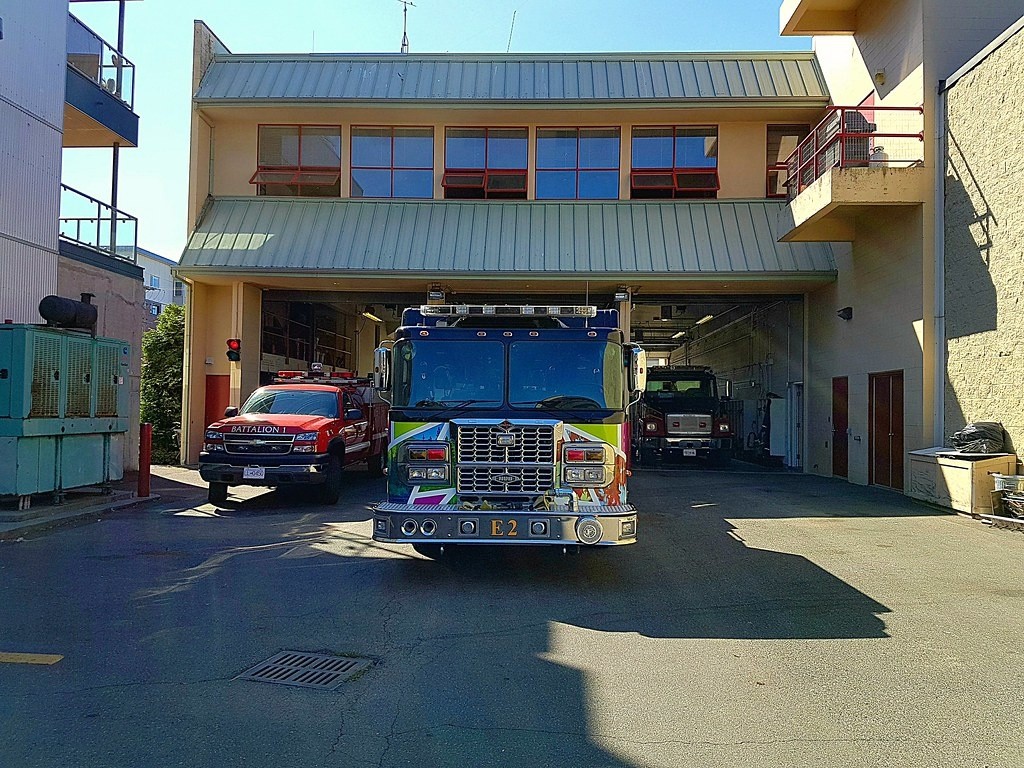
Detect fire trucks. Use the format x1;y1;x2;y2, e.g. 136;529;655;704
630;364;734;469
368;304;648;554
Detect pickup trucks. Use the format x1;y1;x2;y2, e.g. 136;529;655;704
198;367;391;507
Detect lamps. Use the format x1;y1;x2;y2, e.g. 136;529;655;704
428;282;446;301
363;311;383;322
613;284;630;303
874;68;886;84
837;307;853;321
226;338;240;361
